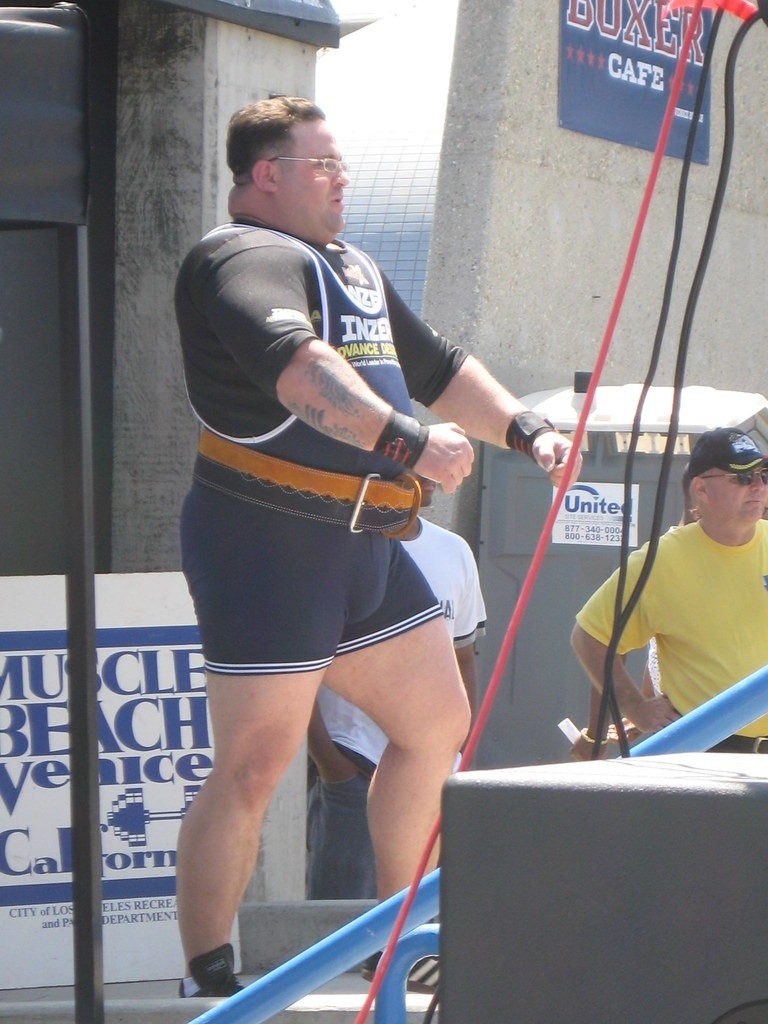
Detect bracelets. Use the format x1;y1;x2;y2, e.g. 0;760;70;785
371;407;429;470
581;728;608;746
505;410;561;464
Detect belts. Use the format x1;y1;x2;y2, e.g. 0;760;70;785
191;419;425;540
719;734;768;754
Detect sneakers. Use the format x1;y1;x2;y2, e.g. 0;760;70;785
362;951;440;994
178;943;242;997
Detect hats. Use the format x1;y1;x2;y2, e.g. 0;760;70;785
688;426;768;479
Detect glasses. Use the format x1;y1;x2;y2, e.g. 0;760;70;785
700;469;768;486
273;155;349;173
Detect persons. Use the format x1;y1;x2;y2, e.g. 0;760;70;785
176;96;584;996
571;427;768;758
